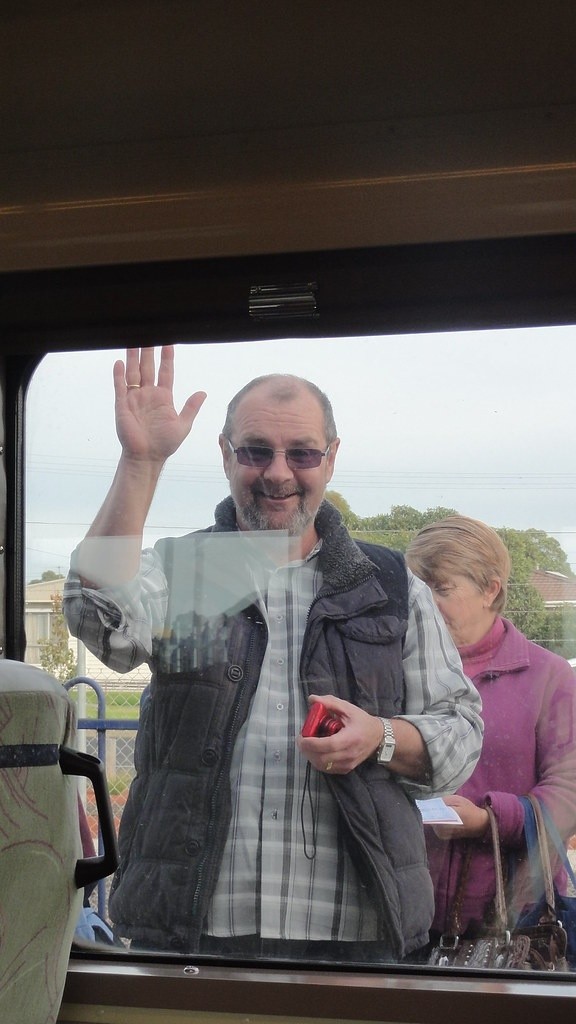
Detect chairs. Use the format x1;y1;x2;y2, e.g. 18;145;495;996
0;660;121;1024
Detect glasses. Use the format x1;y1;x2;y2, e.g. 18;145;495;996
228;441;331;470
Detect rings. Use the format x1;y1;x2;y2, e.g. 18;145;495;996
326;762;333;771
128;385;140;388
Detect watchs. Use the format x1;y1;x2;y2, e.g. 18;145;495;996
366;717;395;765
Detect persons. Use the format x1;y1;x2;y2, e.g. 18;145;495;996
60;345;484;965
405;515;576;965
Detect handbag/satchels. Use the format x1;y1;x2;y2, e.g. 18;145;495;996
427;793;576;973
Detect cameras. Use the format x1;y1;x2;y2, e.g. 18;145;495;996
301;702;343;738
